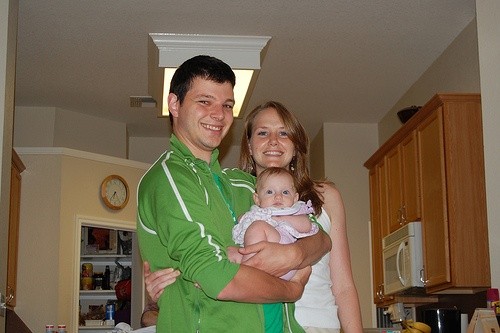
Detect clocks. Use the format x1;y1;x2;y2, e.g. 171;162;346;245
101;175;131;211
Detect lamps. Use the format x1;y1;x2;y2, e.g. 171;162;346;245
148;32;272;117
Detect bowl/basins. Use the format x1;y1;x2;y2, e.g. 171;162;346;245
396;108;420;124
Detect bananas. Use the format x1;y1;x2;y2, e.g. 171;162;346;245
402;319;431;333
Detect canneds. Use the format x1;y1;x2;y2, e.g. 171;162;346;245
105;303;115;320
92;272;104;291
82;263;93;277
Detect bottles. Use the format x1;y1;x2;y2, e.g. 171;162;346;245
80;263;94;290
94;271;102;290
102;265;110;290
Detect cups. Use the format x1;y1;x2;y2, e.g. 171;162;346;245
461;314;468;333
424;308;461;333
58;325;66;333
46;325;54;333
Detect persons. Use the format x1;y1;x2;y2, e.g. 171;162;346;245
137;56;333;333
144;101;364;333
141;302;159;328
193;167;318;288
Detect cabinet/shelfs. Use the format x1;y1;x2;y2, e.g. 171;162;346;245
78;250;133;333
363;93;492;309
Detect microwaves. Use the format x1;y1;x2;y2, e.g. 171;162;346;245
382;221;426;295
